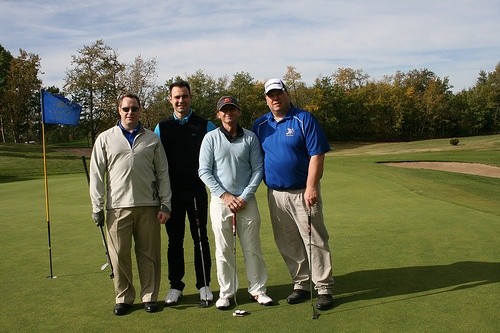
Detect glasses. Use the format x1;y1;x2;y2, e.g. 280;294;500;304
121;107;139;113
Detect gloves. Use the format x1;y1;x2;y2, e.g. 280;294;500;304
92;209;105;227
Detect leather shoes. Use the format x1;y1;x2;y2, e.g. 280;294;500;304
113;303;132;315
143;302;158;313
287;289;311;304
316;294;333;310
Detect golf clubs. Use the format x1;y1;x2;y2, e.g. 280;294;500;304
232;212;246;317
194;197;212;308
307;204;319;319
81;155;109;271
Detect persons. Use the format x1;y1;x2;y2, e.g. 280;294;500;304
251;79;335;311
88;93;173;315
199;96;274;308
153;81;213;306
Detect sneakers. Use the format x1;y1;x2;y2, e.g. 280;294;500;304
250;293;274;306
215;296;231;310
165;288;183;307
199;286;214;303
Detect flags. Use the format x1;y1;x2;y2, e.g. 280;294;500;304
41;90;81;126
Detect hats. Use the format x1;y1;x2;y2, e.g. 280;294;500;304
216;95;238;111
264;78;286;94
